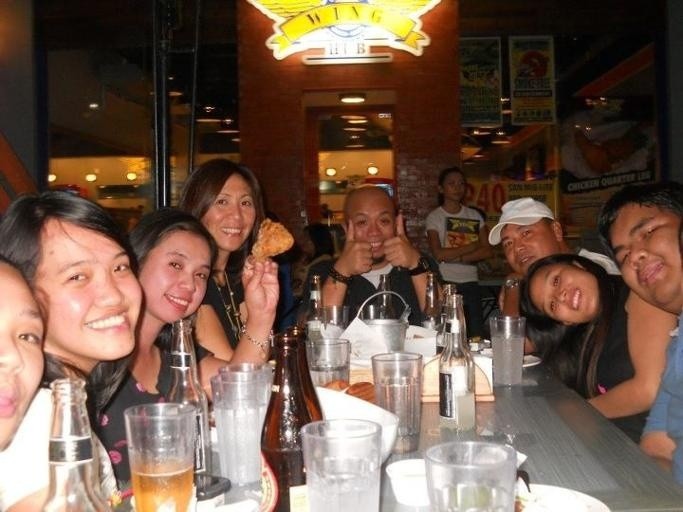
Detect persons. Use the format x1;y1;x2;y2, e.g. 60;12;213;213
598;184;681;476
177;160;293;359
0;259;43;512
7;185;143;512
296;185;441;323
90;205;278;479
488;200;620;384
424;169;490;338
519;253;674;444
291;224;332;314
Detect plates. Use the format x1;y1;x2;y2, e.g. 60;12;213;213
522;354;543;367
515;483;612;512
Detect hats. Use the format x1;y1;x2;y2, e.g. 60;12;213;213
489;197;554;245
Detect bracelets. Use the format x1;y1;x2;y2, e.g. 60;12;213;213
327;267;354;288
238;324;275;360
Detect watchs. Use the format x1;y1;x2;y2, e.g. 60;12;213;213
404;258;429;275
504;278;522;288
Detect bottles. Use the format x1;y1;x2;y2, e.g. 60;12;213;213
166;319;210;493
422;273;441;328
497;279;521;316
44;380;110;512
287;327;323;421
437;284;457;354
304;275;326;333
260;337;314;512
439;296;476;432
376;275;396;320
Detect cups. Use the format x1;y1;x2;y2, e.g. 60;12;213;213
385;459;426;508
322;306;349;328
427;441;515;511
210;372;273;483
124;403;197;512
306;338;350;386
372;352;422;454
489;317;526;386
301;419;381;512
217;364;273;372
